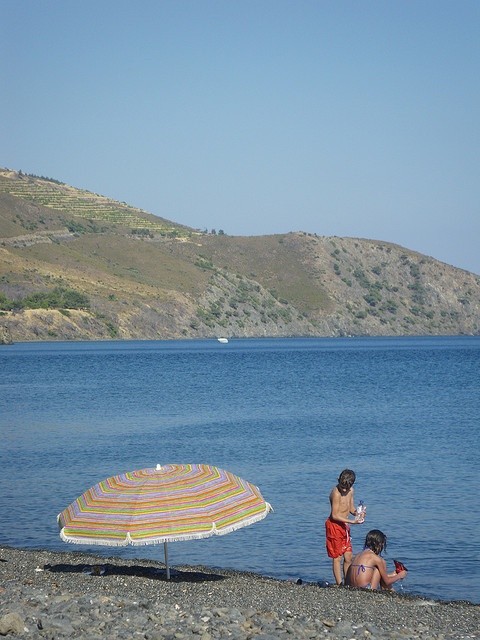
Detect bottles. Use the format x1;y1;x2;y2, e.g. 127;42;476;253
356;501;365;520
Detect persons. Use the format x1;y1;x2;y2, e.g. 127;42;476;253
326;469;366;584
345;528;408;588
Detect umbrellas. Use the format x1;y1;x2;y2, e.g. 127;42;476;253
54;463;275;578
